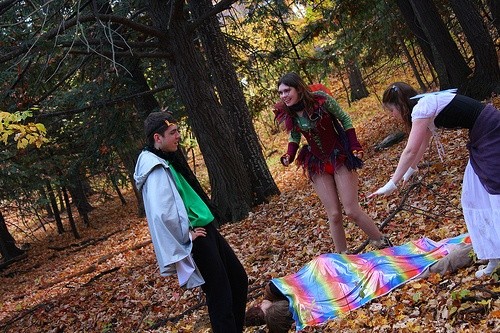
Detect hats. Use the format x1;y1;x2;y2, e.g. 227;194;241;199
143;112;177;140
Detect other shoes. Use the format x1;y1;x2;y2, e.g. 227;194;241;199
474;259;500;279
371;234;394;250
475;258;489;265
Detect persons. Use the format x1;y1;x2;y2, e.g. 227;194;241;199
246;282;295;333
272;71;393;256
366;82;500;279
133;113;248;333
